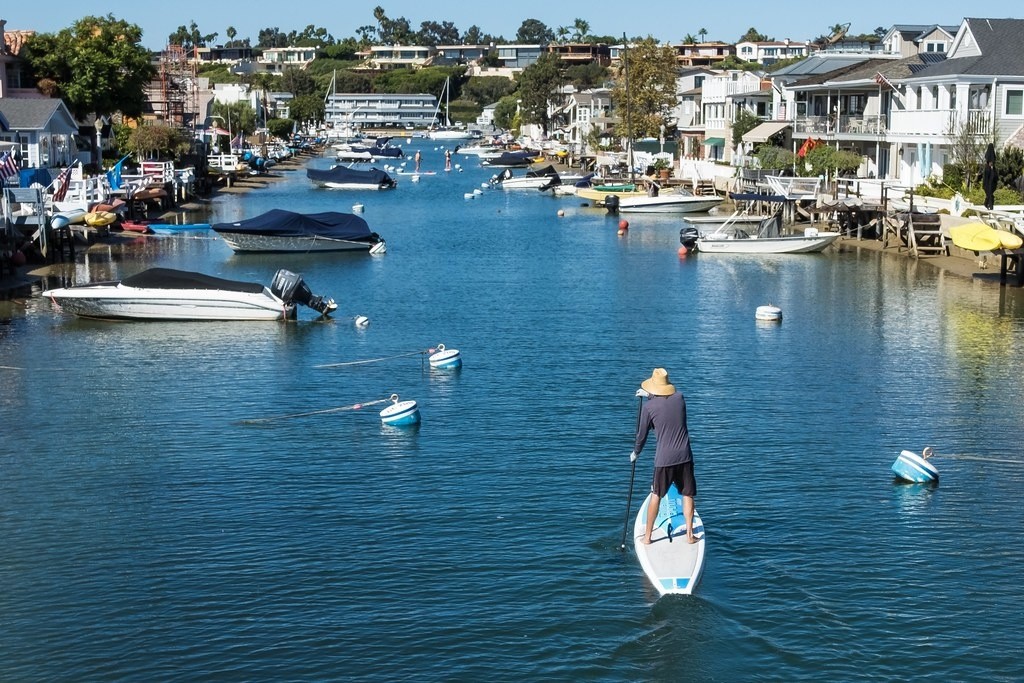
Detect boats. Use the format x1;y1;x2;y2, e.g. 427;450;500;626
211;207;388;255
684;215;772;236
572;174;693;206
147;223;212;237
84;210;117;228
493;168;586;190
118;218;151;233
304;158;397;191
41;267;337;321
948;221;1002;252
133;193;168;200
50;208;89;230
678;210;843;255
456;129;568;167
890;196;938;214
550;182;637;196
993;228;1023;250
89;198;126;219
604;192;726;214
327;106;406;160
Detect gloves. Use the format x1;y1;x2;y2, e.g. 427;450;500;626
636;389;650;398
630;451;638;463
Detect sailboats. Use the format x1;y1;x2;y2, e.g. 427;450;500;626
588;31;716;188
419;75;473;140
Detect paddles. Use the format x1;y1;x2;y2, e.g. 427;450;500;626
621;396;644;551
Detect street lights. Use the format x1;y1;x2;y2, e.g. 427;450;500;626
212;120;218;135
94;118;104;172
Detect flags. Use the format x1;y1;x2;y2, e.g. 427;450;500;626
51;164;72;201
107;156;128;190
0;150;20;181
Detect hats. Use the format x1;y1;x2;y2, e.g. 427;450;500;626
640;368;675;396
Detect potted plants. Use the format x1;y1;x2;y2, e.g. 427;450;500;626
651;156;672;181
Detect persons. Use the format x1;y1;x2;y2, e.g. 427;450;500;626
444;150;452;169
629;367;702;543
414;150;422;171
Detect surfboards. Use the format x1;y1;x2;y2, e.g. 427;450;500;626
633;481;707;597
